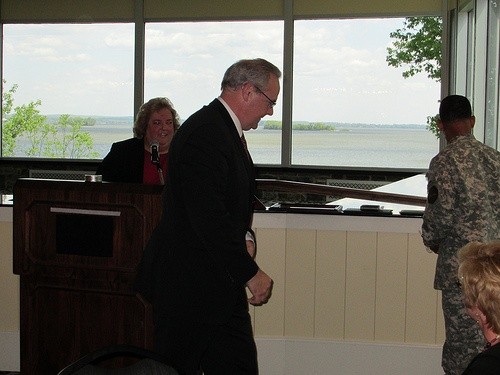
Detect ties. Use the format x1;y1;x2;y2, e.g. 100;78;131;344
240;133;254;168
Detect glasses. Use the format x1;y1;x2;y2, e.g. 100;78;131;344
456;277;464;289
243;83;276;108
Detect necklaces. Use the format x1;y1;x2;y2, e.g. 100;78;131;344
484;335;500;349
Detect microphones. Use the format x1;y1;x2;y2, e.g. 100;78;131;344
150;140;160;168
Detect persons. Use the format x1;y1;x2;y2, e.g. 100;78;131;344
95;97;180;184
421;95;500;375
138;60;280;375
456;240;500;375
